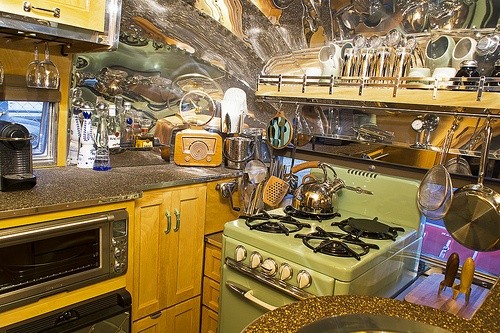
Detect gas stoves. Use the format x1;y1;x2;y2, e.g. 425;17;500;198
223;204;417;289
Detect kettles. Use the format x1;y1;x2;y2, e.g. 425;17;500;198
290;161;345;214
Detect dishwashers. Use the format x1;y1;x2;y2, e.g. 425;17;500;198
218;259;349;333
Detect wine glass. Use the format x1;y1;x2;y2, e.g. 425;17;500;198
35;42;59;89
26;45;45;88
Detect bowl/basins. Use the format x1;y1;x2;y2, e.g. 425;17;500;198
430;68;457;89
407;68;430;88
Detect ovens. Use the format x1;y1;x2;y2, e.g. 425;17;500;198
0;208;131;333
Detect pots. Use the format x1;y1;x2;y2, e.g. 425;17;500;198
443;126;500;252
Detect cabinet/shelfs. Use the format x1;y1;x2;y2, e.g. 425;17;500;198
0;0;241;330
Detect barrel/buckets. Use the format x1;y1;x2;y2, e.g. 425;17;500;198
222;137;256;170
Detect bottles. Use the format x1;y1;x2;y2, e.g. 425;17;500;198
66;101;134;171
